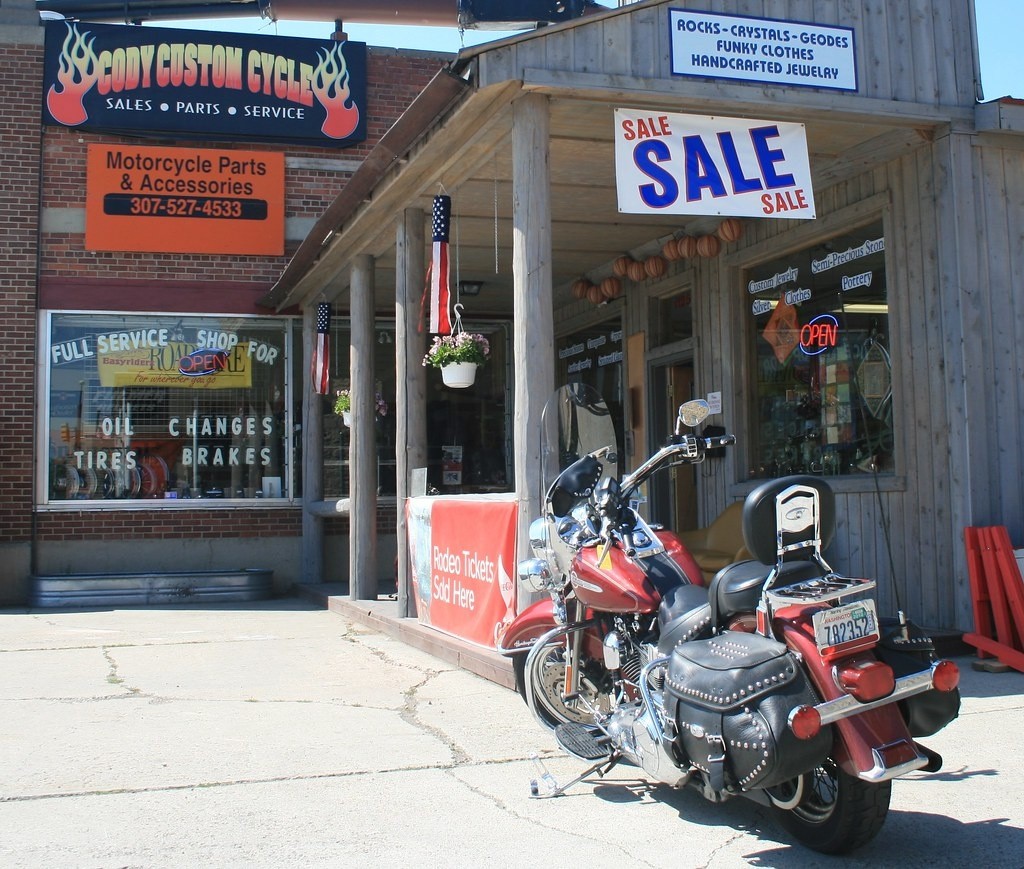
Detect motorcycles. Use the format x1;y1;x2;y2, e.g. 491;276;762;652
498;394;961;854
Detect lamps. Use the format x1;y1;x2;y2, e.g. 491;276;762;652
455;280;483;296
377;331;392;343
597;294;625;308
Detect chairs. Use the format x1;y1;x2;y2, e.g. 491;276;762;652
675;501;754;586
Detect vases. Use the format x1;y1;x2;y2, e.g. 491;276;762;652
341;413;350;427
440;360;477;388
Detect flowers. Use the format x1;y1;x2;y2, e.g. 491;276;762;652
422;331;490;367
334;390;388;415
